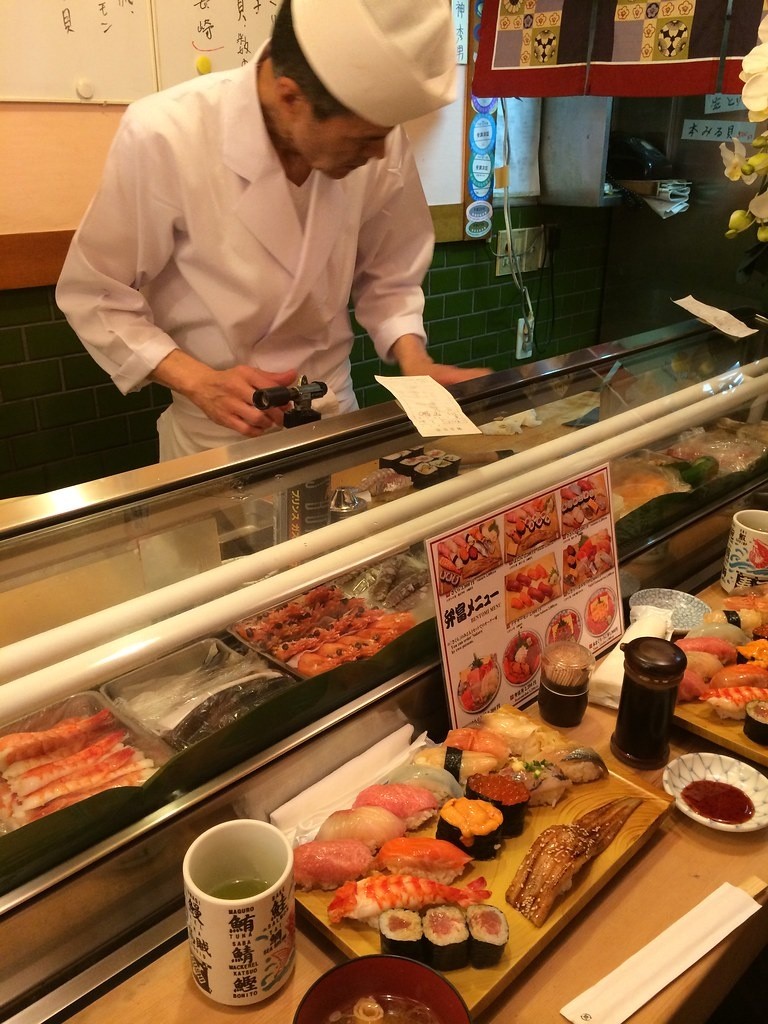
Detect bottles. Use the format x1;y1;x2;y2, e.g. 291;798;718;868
610;637;689;770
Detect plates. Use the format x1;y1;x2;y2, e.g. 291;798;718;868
663;751;768;834
457;656;502;713
545;605;584;648
503;629;544;686
628;587;714;636
584;587;618;637
0;553;434;836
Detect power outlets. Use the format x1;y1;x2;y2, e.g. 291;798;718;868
515;321;535;360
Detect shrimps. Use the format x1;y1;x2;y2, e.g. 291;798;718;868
327;876;493;926
232;585;415;679
0;709;162;837
698;685;768;713
439;557;462;575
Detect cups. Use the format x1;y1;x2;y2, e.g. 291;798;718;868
718;506;767;594
537;666;592;729
182;818;299;1008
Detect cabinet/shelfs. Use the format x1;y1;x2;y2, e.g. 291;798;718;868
539;96;674;208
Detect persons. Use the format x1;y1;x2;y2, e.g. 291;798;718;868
53;1;496;463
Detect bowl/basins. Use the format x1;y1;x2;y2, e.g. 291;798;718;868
288;951;475;1024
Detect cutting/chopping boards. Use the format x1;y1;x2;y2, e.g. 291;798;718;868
669;681;767;770
275;710;670;1012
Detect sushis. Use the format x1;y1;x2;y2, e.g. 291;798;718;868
294;705;644;970
675;594;768;744
379;445;461;488
436;475;615;610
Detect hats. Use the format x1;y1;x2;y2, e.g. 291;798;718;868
291;0;459;128
